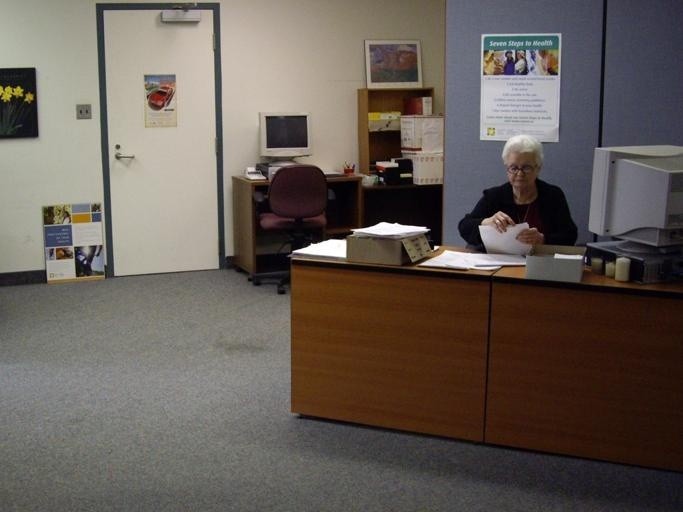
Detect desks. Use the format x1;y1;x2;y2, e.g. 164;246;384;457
231;172;365;283
287;237;504;442
484;265;682;473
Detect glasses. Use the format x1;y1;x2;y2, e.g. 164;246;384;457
507;163;536;174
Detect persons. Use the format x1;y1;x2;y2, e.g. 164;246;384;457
457;133;578;254
49;248;54;258
503;49;548;75
63;211;71;224
92;204;101;212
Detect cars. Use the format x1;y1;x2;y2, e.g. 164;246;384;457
148;86;172;109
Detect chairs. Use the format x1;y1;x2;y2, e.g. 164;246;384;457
251;165;329;294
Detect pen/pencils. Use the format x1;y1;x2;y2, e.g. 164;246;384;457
344;160;355;169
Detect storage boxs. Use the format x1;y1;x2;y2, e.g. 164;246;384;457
346;233;428;265
401;114;445;154
401;153;444;185
402;96;433;115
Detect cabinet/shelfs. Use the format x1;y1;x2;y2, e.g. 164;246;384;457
358;86;446;247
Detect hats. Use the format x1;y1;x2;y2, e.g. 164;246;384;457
517;50;525;57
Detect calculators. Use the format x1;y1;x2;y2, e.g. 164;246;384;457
244;167;267;180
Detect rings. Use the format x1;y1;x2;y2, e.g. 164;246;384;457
495;220;499;223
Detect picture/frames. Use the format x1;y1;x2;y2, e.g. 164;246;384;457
363;38;424;90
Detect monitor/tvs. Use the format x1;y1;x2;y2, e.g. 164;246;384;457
587;145;683;255
258;110;312;166
